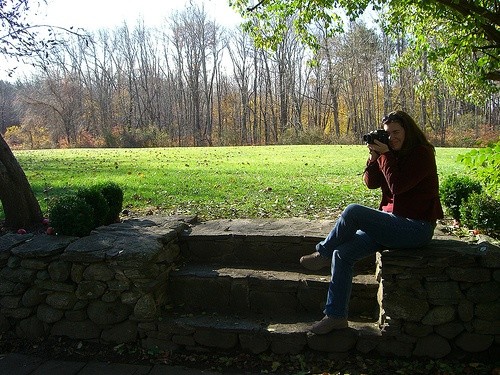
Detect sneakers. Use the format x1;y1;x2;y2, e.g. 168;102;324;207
300;252;330;270
310;315;348;335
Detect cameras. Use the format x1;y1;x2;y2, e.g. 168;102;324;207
364;129;389;146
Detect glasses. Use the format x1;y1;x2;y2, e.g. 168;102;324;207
382;113;402;121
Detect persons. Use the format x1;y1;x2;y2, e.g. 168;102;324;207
300;111;444;334
